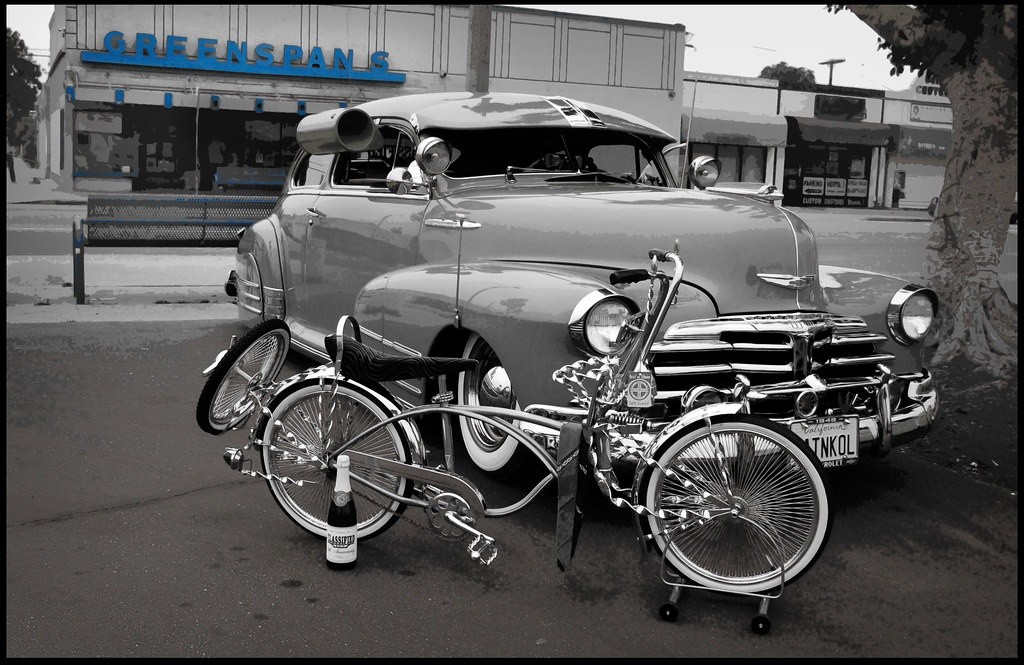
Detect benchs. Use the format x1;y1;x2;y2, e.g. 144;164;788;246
72;193;280;305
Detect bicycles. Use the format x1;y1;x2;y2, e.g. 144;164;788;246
196;245;833;596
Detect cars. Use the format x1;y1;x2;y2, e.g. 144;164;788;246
224;89;943;492
